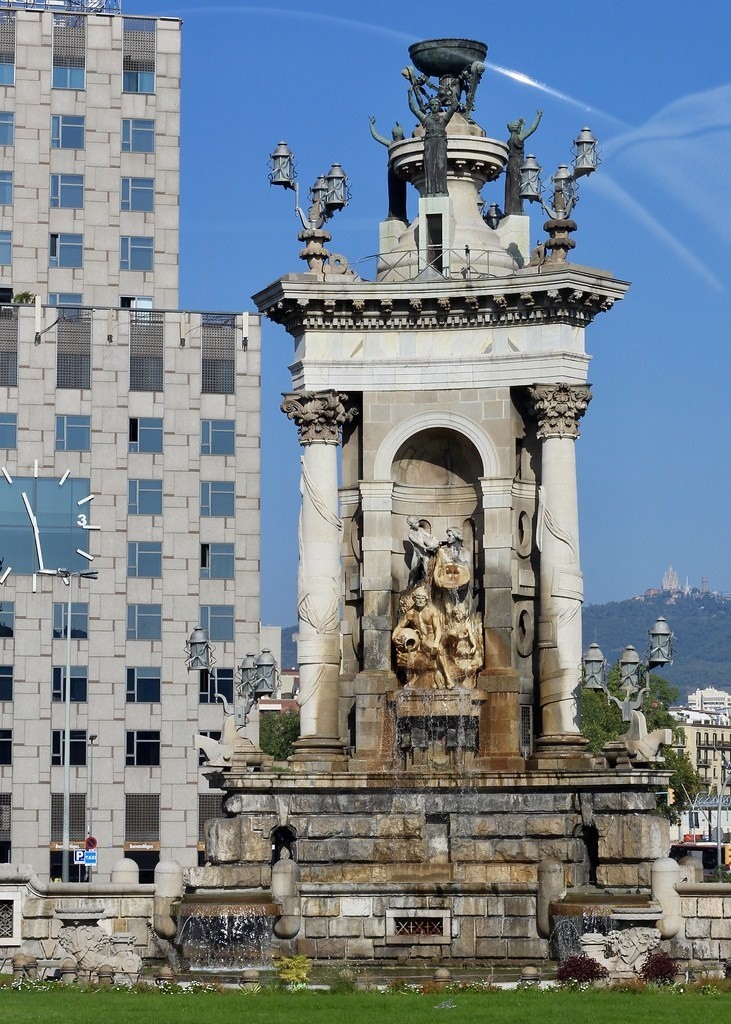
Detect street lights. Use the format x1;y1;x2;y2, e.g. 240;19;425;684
89;733;98;839
61;570;100;887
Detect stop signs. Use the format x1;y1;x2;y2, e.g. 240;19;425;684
74;849;87;865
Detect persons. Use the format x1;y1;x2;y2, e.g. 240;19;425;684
368;83;458;220
505;109;543;215
393;515;484;690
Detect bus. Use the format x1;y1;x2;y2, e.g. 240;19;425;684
668;843;731;881
